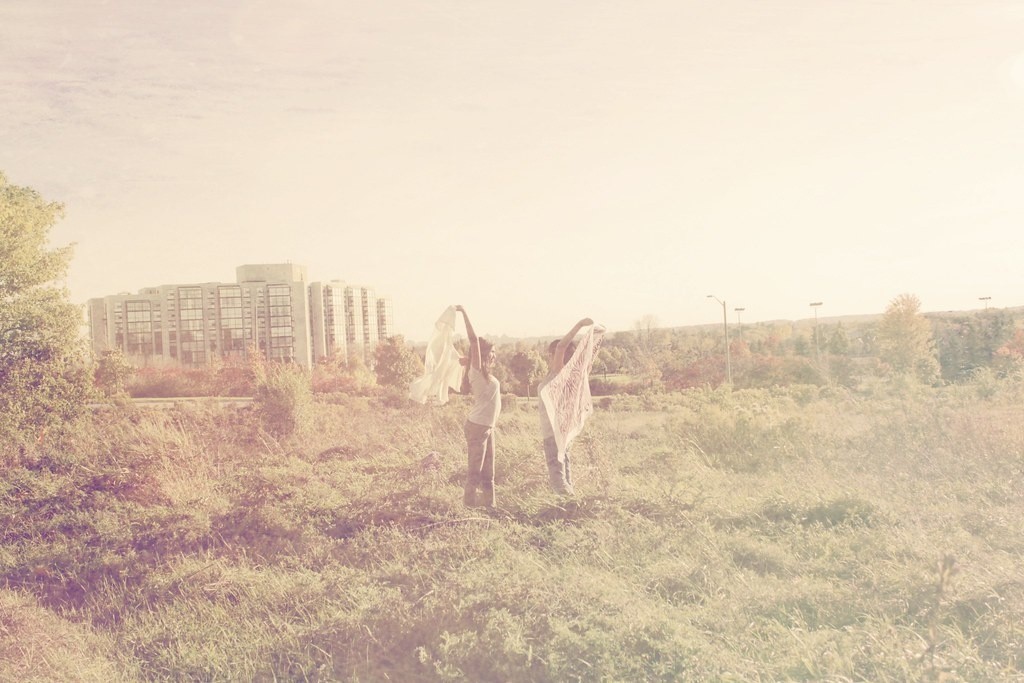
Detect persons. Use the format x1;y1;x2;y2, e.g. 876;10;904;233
537;317;595;498
455;305;501;511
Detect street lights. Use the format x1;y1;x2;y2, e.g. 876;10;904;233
979;297;992;319
735;307;744;345
707;294;731;384
809;302;824;354
